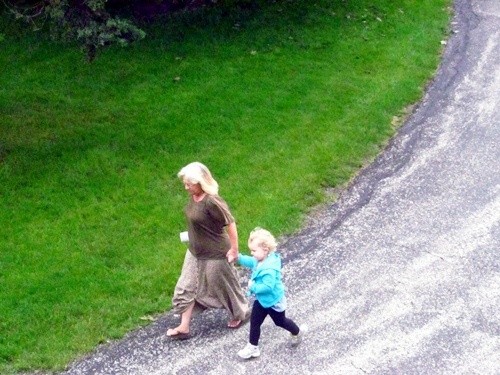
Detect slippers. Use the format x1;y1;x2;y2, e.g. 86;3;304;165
166;330;189;337
227;319;242;328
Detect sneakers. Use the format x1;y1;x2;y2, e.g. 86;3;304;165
291;331;301;344
237;344;260;359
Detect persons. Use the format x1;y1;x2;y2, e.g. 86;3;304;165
166;161;251;339
227;226;306;359
